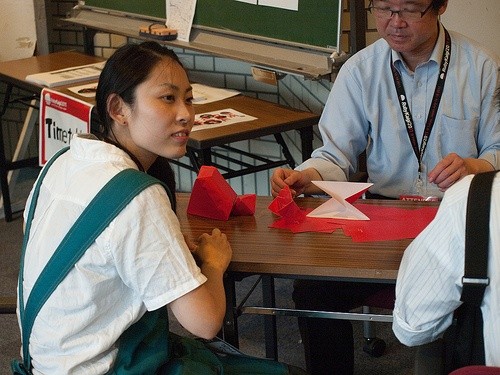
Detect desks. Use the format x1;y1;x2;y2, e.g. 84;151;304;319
0;48;321;225
176;191;441;362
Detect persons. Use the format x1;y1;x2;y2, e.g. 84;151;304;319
9;40;314;375
270;0;500;375
390;170;500;374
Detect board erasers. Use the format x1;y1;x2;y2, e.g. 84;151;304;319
138;21;179;42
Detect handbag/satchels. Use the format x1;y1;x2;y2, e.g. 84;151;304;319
442;305;486;375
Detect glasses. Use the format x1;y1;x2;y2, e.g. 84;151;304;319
368;0;434;21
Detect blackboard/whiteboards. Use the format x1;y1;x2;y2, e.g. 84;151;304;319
64;0;343;79
